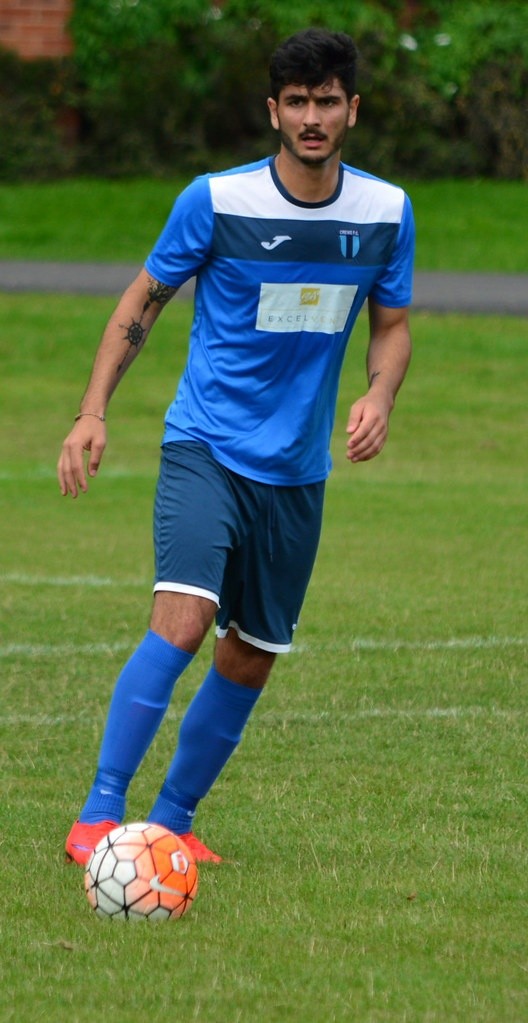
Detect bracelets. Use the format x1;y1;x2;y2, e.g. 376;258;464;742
74;413;106;422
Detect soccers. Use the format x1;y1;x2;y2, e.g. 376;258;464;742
84;822;199;923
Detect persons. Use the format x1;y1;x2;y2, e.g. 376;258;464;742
57;31;414;864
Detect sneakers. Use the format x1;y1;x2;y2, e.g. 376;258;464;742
65;819;120;868
179;832;223;862
